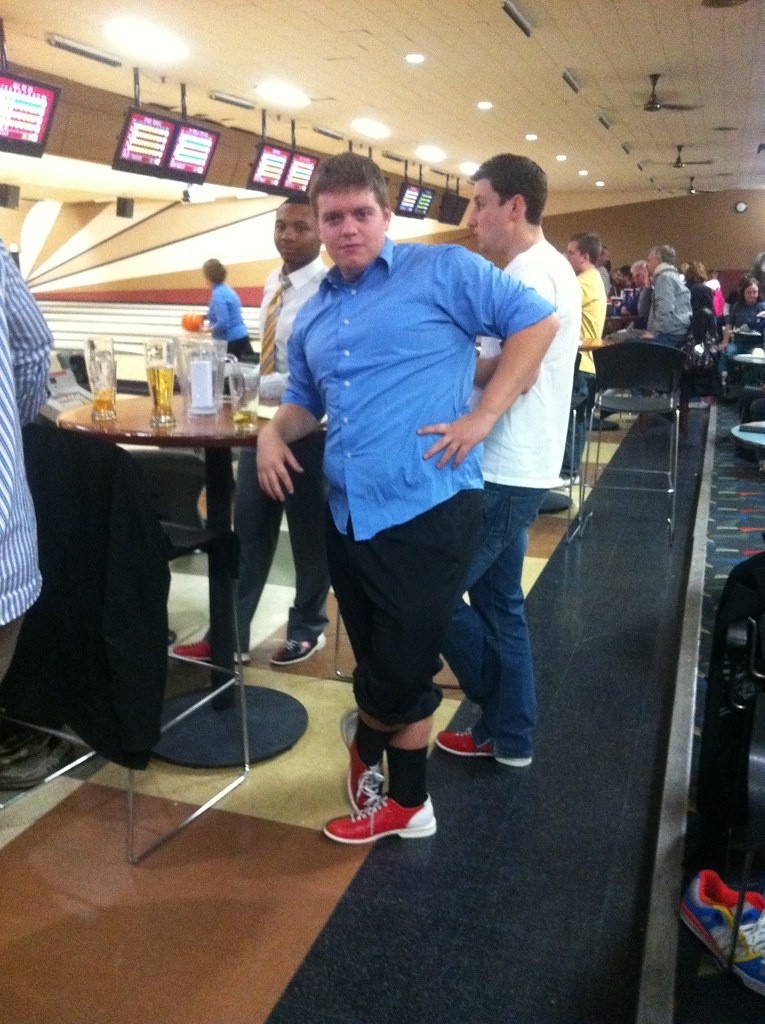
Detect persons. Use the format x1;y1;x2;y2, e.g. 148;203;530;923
435;154;582;767
257;152;560;845
172;190;333;665
198;259;254;353
596;244;765;408
561;232;606;484
0;236;53;684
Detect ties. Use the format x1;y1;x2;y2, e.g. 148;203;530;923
260;282;283;375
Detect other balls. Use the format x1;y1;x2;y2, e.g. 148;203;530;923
182;312;204;332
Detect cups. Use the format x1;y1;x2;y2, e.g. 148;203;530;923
229;376;261;430
83;337;116;419
145;338;179;428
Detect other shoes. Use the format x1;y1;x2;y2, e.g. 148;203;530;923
559;477;580;491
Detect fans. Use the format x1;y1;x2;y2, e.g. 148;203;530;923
670;176;717;196
651;145;710;167
599;74;703;114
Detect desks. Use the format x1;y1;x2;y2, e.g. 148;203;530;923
573;331;632;351
729;420;765;458
734;328;764;341
734;350;764;365
56;397;313;771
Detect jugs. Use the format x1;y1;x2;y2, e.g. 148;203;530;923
171;337;244;420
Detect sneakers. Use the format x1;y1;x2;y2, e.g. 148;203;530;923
173;635;252;663
679;869;765;997
324;793;437;843
271;629;328;666
339;707;385;810
435;726;533;768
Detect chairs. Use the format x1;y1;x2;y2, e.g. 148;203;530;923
0;292;691;869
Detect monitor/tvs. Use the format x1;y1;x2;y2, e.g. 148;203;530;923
111;107;470;227
0;70;60;158
